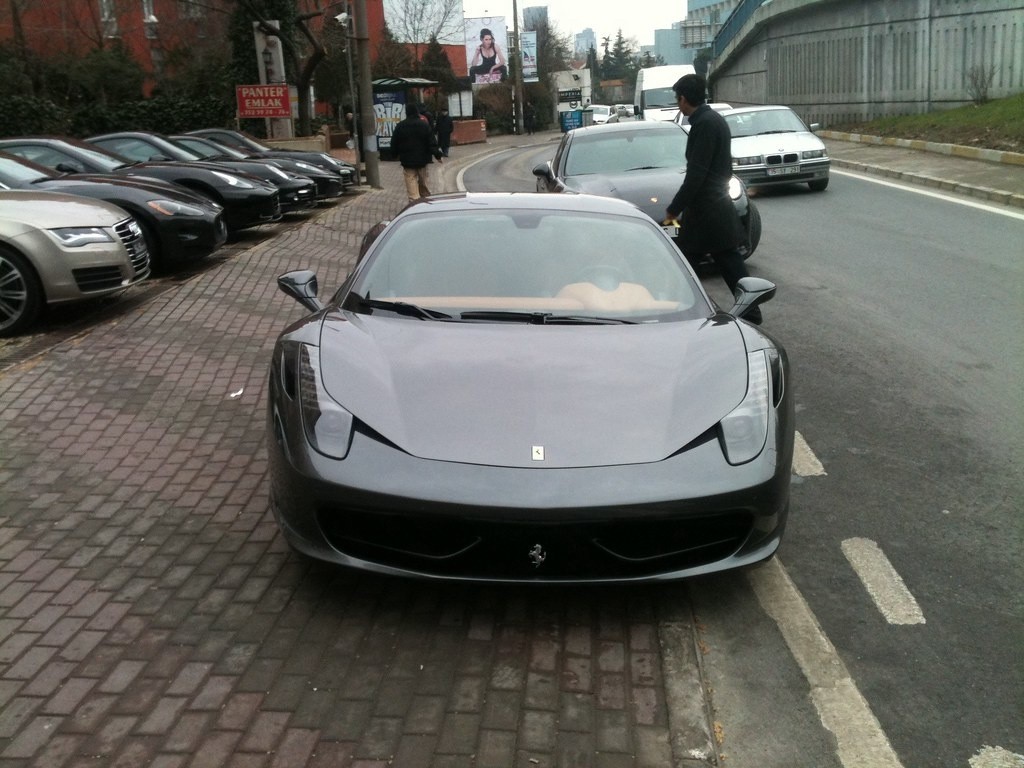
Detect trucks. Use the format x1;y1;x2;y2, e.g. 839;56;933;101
633;64;710;123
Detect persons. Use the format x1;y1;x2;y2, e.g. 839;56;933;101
666;74;764;326
415;104;438;163
391;103;437;204
347;113;366;162
470;28;507;84
435;108;453;157
524;102;536;136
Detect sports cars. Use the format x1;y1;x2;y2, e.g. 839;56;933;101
266;193;794;586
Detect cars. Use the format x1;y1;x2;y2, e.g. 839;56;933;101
0;151;228;279
0;135;284;231
672;103;830;195
0;190;151;338
533;120;762;270
82;127;356;211
584;104;618;125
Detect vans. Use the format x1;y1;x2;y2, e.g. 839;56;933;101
614;104;635;117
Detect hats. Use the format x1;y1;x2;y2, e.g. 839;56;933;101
442;106;449;111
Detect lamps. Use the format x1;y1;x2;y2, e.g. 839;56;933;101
573;74;580;81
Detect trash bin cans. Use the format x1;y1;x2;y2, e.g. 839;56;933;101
576;109;593;126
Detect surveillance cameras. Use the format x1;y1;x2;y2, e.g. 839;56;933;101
334;12;347;23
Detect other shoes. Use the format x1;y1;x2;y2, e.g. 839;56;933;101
740;305;762;325
444;152;448;157
